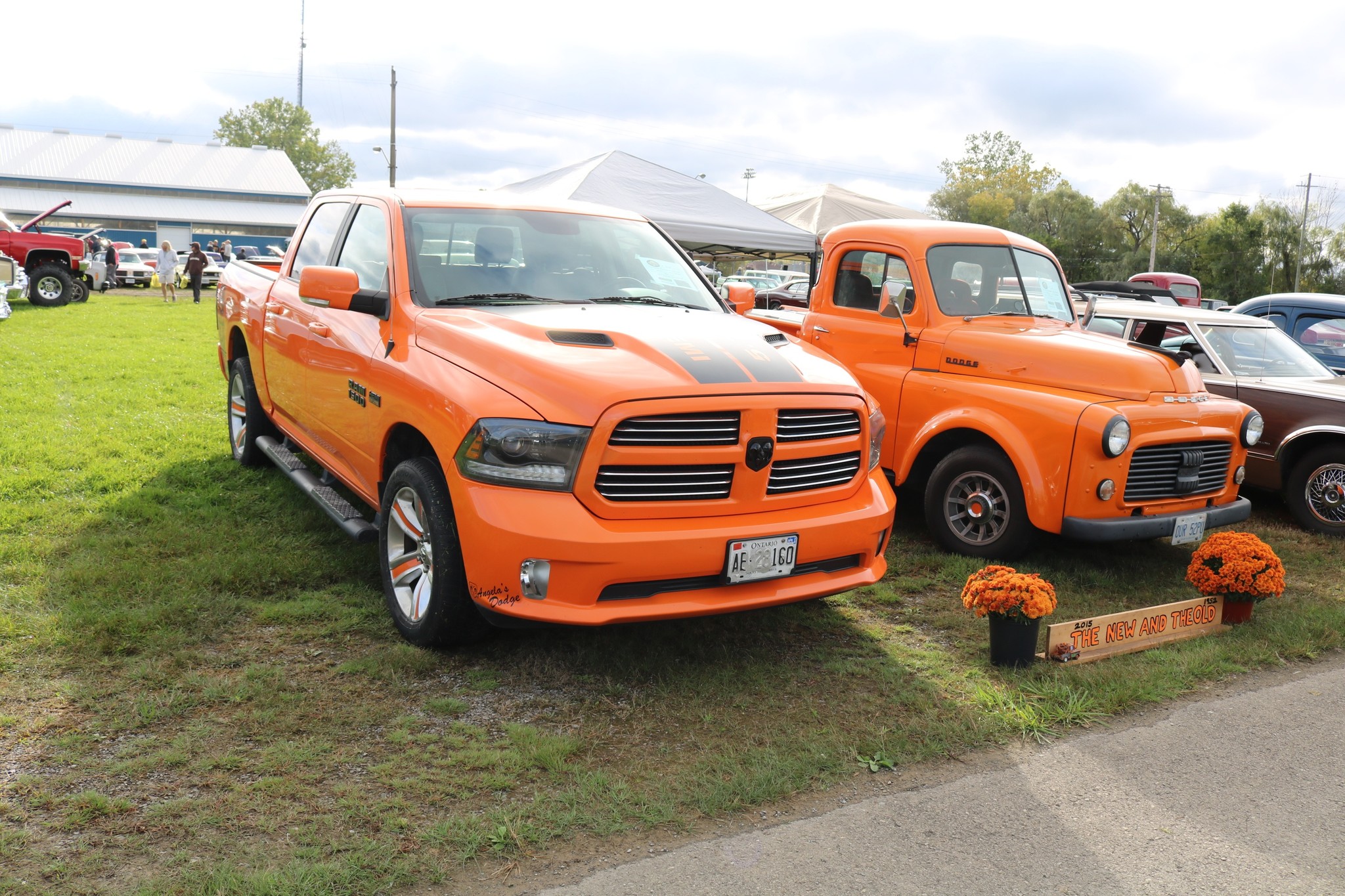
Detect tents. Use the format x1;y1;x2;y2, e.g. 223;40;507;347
746;182;939;262
494;150;817;304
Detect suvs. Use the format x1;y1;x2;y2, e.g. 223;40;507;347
0;200;89;307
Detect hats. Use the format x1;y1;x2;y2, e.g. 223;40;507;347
189;243;195;246
224;240;231;243
98;237;102;240
209;241;214;244
141;239;147;242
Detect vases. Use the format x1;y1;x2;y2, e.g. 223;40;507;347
1221;597;1254;626
988;614;1042;668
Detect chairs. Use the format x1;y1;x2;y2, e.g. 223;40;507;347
834;273;980;317
412;224;448;301
510;217;601;298
466;227;517;293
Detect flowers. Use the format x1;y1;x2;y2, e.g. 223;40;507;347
1185;530;1286;605
960;565;1057;626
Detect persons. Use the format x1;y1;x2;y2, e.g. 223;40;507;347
736;267;743;275
84;237;245;305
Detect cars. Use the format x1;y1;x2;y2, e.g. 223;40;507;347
715;268;810;310
71;228;284;311
971;271;1345;538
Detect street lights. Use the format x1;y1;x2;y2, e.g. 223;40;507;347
373;147;396;188
742;168;756;202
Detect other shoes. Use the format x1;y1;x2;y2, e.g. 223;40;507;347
118;282;124;288
172;294;177;302
163;299;169;302
112;282;117;288
195;301;200;304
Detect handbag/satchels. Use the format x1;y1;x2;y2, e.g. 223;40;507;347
179;275;188;289
151;273;161;287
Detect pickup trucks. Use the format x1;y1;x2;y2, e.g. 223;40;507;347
216;189;876;658
743;219;1260;563
